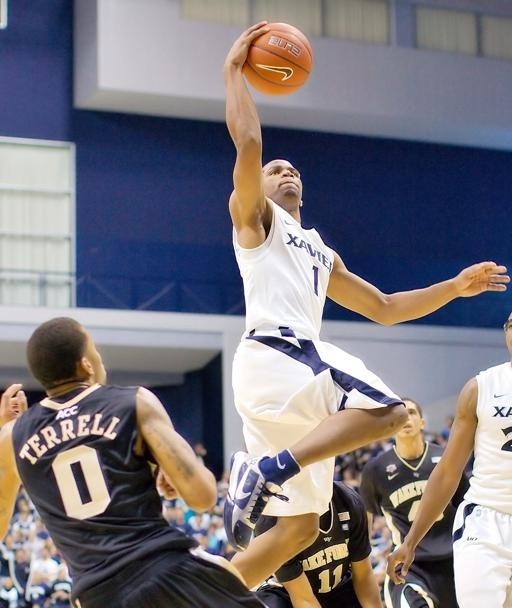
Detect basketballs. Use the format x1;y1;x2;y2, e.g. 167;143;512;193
242;22;313;96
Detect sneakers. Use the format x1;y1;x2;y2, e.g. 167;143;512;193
223;451;290;552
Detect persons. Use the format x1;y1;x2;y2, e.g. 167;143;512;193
334;437;396;607
441;415;454;439
358;398;471;608
221;20;511;590
0;482;240;608
0;316;288;608
387;308;512;608
246;478;385;607
434;435;446;447
